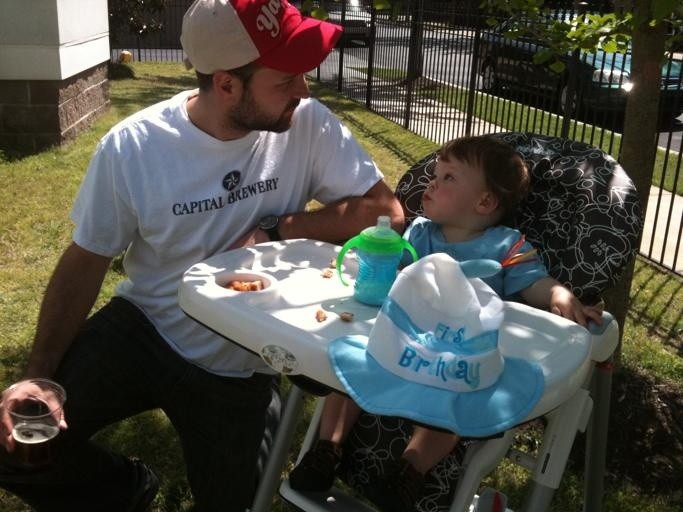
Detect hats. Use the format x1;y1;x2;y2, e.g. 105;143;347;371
179;0;346;76
328;252;546;437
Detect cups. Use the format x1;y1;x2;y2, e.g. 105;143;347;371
5;378;68;468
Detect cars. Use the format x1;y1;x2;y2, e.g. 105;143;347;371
330;9;376;47
479;12;682;121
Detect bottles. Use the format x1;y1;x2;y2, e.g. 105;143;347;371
335;215;419;306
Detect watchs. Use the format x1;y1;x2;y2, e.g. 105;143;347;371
257;214;283;242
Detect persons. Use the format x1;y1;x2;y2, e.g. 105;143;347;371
0;0;408;511
289;135;606;512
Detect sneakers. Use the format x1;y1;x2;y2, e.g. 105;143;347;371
288;440;344;493
132;457;159;511
364;457;425;511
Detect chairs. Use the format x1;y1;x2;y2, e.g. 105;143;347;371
176;136;645;512
